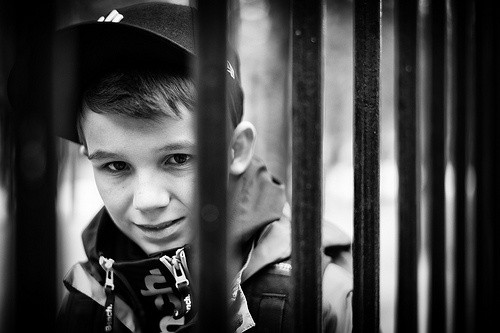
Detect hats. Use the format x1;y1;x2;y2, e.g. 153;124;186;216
7;2;244;147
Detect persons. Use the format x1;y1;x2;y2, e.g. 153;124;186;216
43;2;355;332
